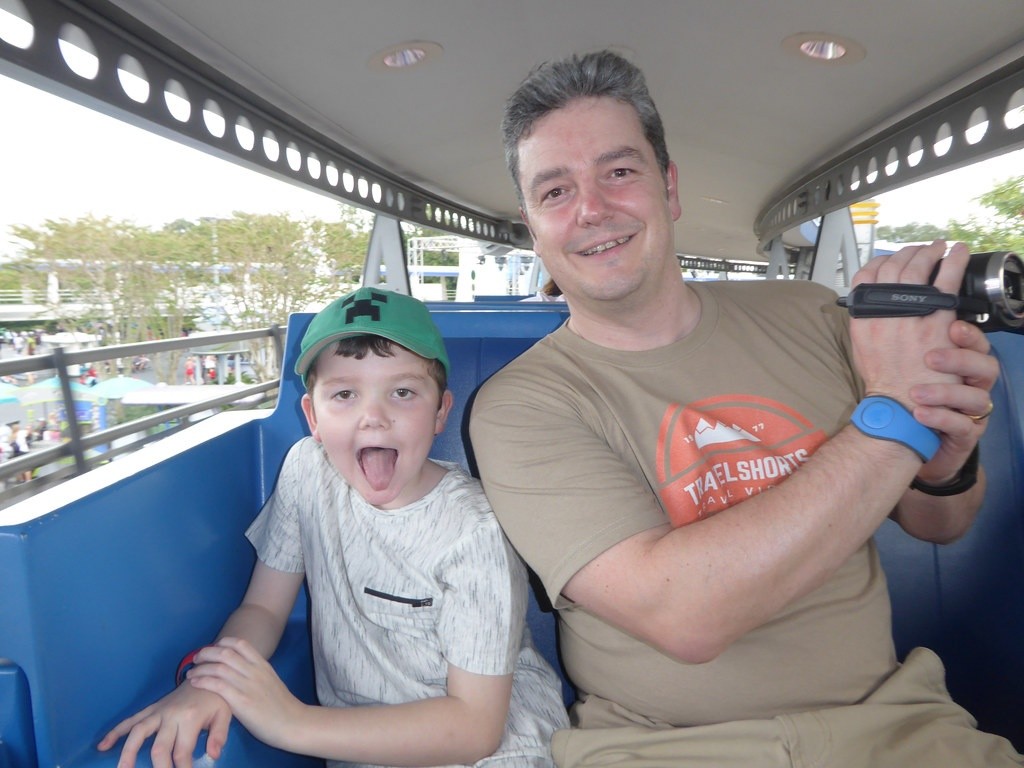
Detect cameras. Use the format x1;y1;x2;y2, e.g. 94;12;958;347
930;250;1024;334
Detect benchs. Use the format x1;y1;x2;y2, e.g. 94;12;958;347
255;295;1024;753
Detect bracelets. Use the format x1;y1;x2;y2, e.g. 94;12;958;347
850;396;941;461
177;643;213;686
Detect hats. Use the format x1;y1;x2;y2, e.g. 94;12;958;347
293;286;452;387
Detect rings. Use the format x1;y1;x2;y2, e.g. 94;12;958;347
967;400;994;420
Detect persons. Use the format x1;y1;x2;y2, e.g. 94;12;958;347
466;51;1024;768
98;287;570;768
0;325;237;483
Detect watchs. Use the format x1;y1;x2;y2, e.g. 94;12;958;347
909;440;980;496
